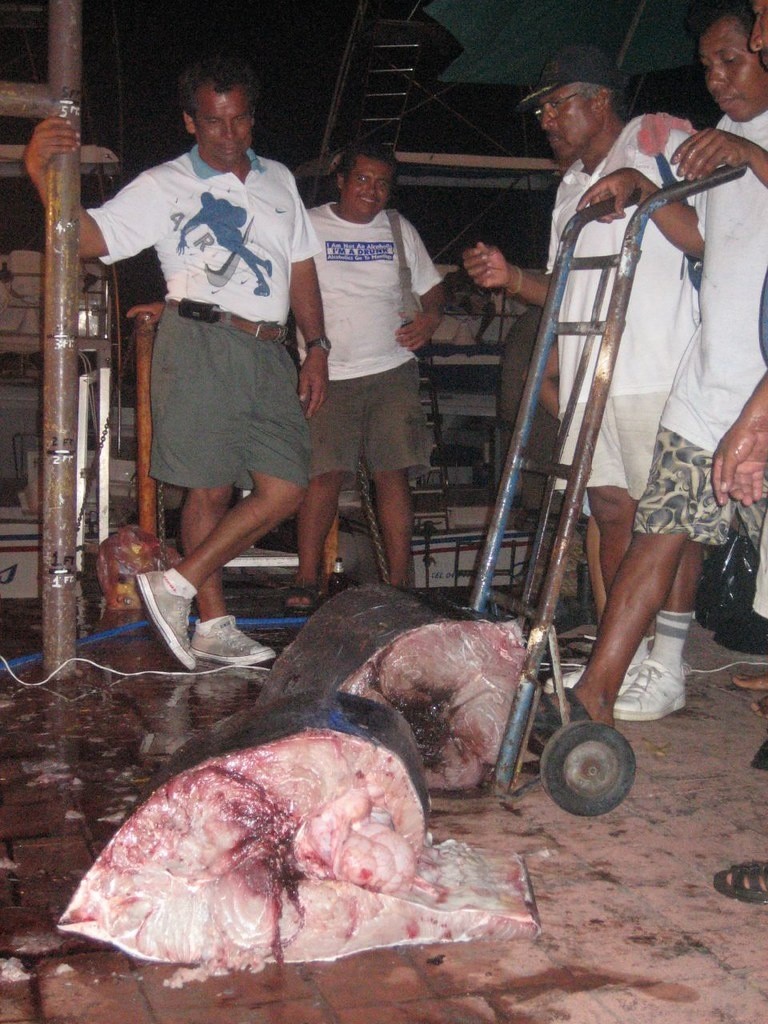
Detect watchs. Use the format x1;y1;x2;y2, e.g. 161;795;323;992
305;336;331;354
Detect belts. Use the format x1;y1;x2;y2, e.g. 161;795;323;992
168;299;286;341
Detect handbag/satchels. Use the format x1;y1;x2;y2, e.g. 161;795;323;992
387;208;424;322
695;508;768;655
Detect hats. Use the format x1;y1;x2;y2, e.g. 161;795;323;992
516;46;612;113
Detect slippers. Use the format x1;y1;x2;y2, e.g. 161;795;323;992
528;688;592;730
285;583;320;613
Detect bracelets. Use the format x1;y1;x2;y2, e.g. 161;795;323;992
506;265;523;294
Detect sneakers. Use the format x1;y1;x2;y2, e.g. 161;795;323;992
188;615;276;666
137;571;197;670
543;655;649;696
613;659;686;721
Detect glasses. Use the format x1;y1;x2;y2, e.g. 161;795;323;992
533;86;592;121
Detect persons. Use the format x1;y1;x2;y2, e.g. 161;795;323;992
24;56;331;670
125;142;446;613
463;0;768;904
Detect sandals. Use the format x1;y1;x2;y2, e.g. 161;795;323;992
714;862;768;903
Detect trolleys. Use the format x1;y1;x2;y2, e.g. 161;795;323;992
425;163;751;821
